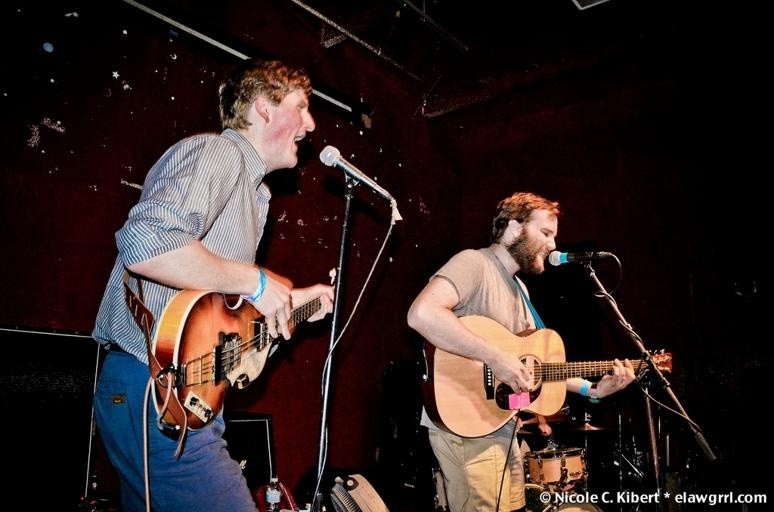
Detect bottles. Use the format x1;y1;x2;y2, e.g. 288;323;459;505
265;478;282;512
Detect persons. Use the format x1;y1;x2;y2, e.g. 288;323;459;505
407;190;637;512
90;61;337;512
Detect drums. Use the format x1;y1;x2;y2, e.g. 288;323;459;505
525;492;601;512
526;446;589;484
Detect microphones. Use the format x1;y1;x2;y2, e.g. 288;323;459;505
320;144;395;204
549;252;621;267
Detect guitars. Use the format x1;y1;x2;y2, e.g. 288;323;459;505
419;315;672;438
151;288;333;433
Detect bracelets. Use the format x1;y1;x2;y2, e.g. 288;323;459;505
579;380;592;396
238;267;266;304
590;383;598;400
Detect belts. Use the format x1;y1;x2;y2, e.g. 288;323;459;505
109;343;126;352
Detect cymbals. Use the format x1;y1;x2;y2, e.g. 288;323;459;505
569;424;604;430
